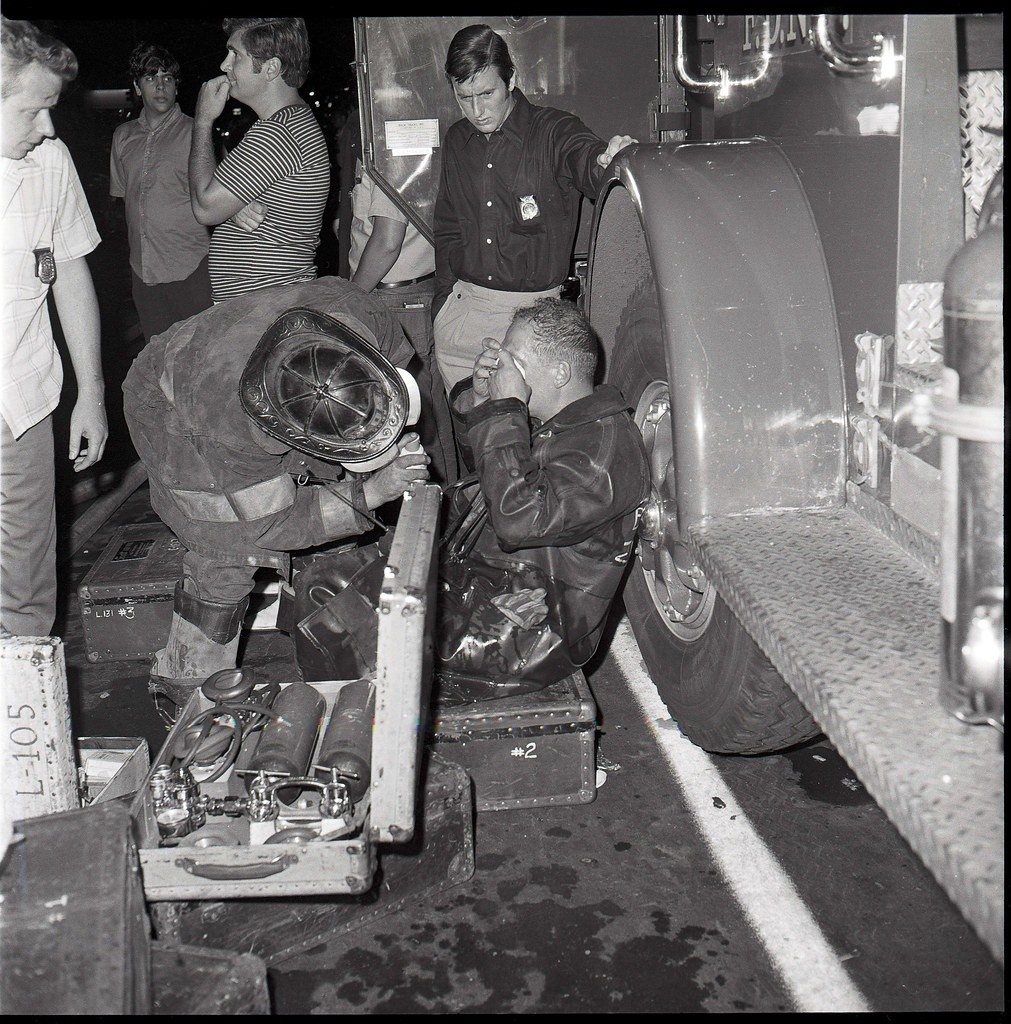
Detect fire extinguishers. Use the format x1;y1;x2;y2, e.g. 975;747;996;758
310;679;375;820
232;682;327;821
936;119;1007;731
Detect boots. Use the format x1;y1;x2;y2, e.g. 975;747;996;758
149;579;251;731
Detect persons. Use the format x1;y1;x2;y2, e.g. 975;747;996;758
189;18;330;306
429;24;638;403
109;42;213;345
333;173;459;490
121;275;432;706
292;298;652;706
0;14;108;638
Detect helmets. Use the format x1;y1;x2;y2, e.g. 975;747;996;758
238;307;410;462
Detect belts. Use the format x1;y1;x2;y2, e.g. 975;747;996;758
376;271;434;290
